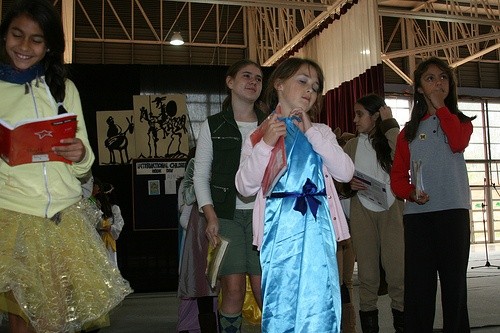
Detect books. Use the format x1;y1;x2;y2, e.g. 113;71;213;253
356;167;388;211
205;232;230;289
0;113;78;167
411;159;424;200
250;104;287;199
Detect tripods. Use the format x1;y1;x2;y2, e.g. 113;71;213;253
471;177;500;269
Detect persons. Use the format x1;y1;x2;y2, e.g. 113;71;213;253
86;176;124;265
342;94;404;333
391;57;477;333
193;59;266;333
0;0;134;333
235;56;355;333
178;150;217;332
